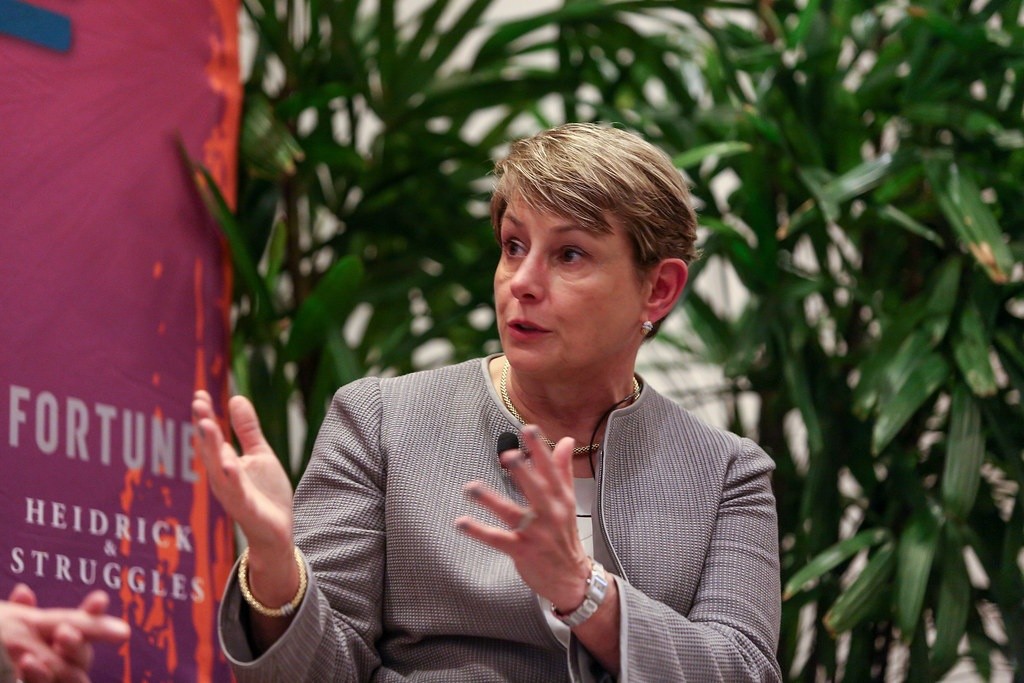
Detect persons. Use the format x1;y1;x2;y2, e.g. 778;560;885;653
0;583;130;683
193;123;781;683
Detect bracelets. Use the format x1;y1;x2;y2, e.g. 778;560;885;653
237;545;305;616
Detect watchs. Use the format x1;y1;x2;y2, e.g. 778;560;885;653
551;555;607;626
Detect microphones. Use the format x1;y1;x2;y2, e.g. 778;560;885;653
497;432;519;469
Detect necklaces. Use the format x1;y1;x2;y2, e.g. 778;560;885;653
502;360;640;456
